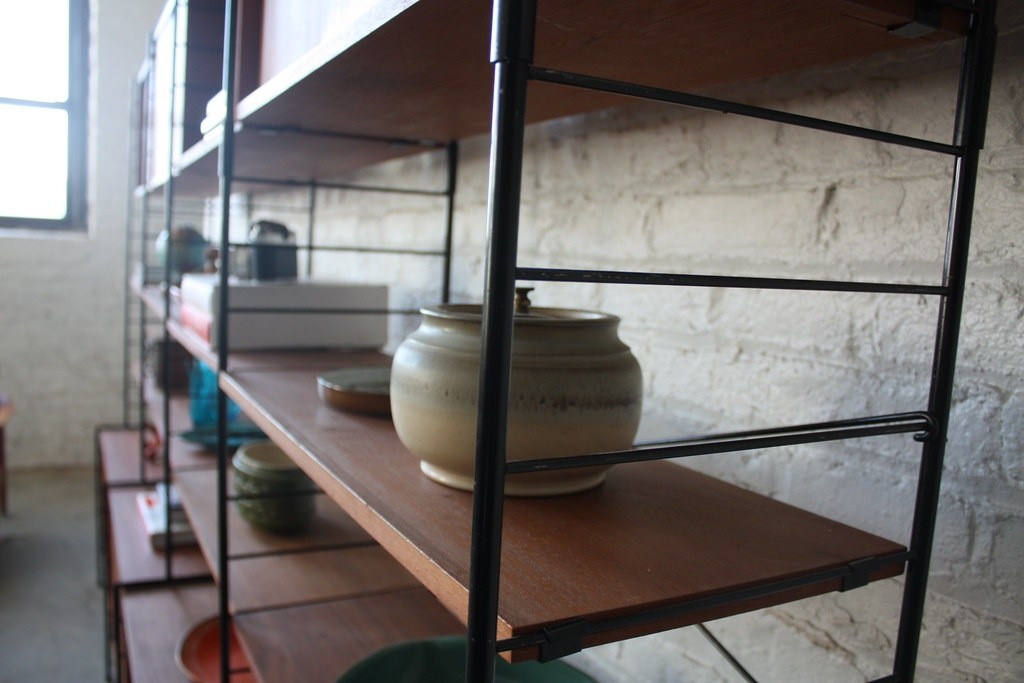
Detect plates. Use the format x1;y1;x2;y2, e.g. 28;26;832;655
175;613;260;683
337;636;600;683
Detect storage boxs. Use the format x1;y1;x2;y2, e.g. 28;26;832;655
157;483;181;511
138;490;196;549
181;272;389;351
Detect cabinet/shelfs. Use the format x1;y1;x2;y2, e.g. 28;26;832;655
92;0;999;683
